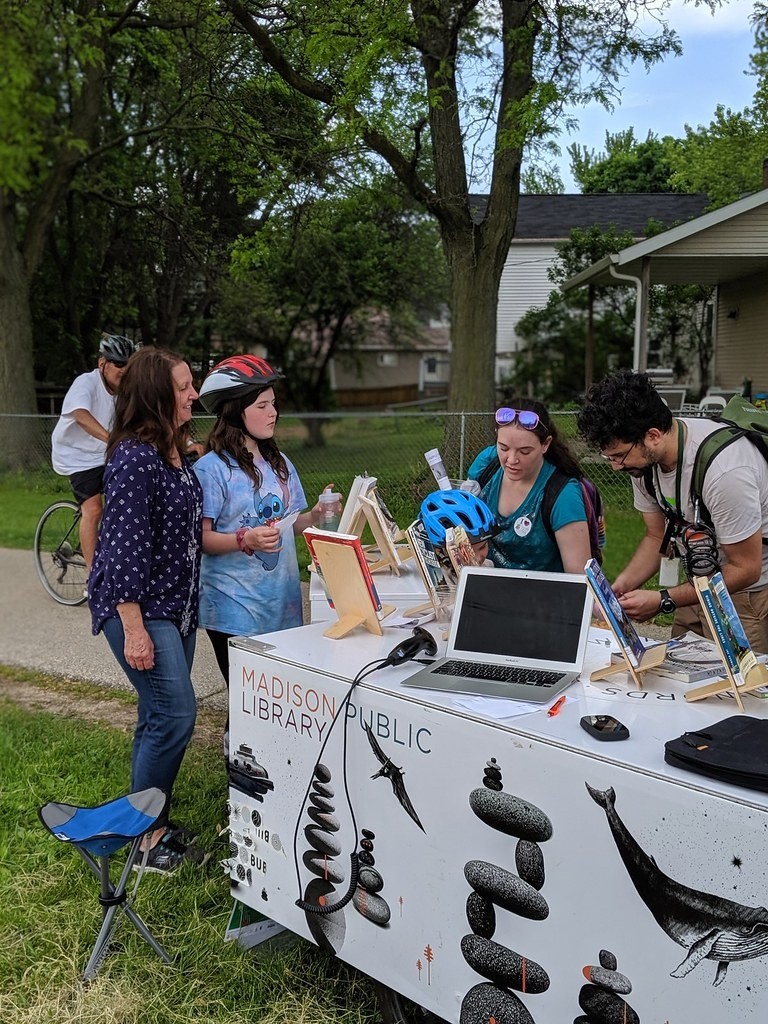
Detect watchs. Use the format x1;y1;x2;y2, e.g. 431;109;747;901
659;589;677;615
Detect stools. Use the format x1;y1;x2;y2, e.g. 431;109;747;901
36;787;174;983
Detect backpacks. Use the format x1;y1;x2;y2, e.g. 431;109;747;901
643;394;768;546
477;454;605;568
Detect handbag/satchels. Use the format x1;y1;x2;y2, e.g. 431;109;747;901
665;715;768;793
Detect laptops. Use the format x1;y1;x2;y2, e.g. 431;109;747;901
401;566;594;704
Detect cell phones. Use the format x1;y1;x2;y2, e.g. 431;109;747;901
580;715;631;742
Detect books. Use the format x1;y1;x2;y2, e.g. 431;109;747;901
697;572;758;687
358;487;403;566
611;630;767;683
408;520;460;609
303;527;384;620
584;558;646;669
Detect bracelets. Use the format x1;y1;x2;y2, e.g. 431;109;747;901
235;526;255;557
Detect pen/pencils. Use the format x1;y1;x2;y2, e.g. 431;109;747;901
546;696;566;718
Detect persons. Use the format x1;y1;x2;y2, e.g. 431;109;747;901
195;354;344;756
50;335;143;603
87;351;212;878
467;399;592;574
417;488;502;590
576;370;768;657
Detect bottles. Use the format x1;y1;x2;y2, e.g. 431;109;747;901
319;488;341;534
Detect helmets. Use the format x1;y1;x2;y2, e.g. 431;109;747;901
99;335;137;363
416;490;501;548
198;355;286;415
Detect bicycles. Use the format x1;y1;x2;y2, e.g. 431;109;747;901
33;450;200;608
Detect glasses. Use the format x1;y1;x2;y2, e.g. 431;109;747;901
599;441;639;465
107;360;127;368
495;408;549;433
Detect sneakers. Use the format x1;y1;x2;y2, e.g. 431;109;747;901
128;820;212;878
